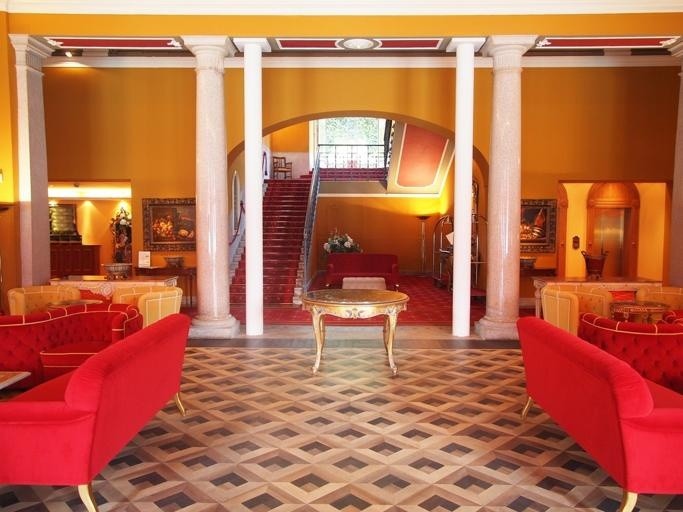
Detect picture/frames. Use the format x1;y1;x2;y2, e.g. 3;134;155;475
519;198;557;254
141;196;196;253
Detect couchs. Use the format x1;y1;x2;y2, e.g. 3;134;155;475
1;311;191;512
3;303;142;385
518;283;683;512
325;252;399;291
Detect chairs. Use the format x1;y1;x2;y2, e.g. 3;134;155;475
7;284;103;312
112;284;184;327
270;156;293;180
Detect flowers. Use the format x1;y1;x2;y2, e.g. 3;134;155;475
319;225;364;258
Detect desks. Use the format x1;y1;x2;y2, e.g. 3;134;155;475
528;274;663;318
299;290;412;377
133;266;197;310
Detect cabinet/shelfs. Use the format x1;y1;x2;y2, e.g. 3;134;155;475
50;244;103;278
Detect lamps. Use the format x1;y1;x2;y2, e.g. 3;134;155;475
413;214;430;277
